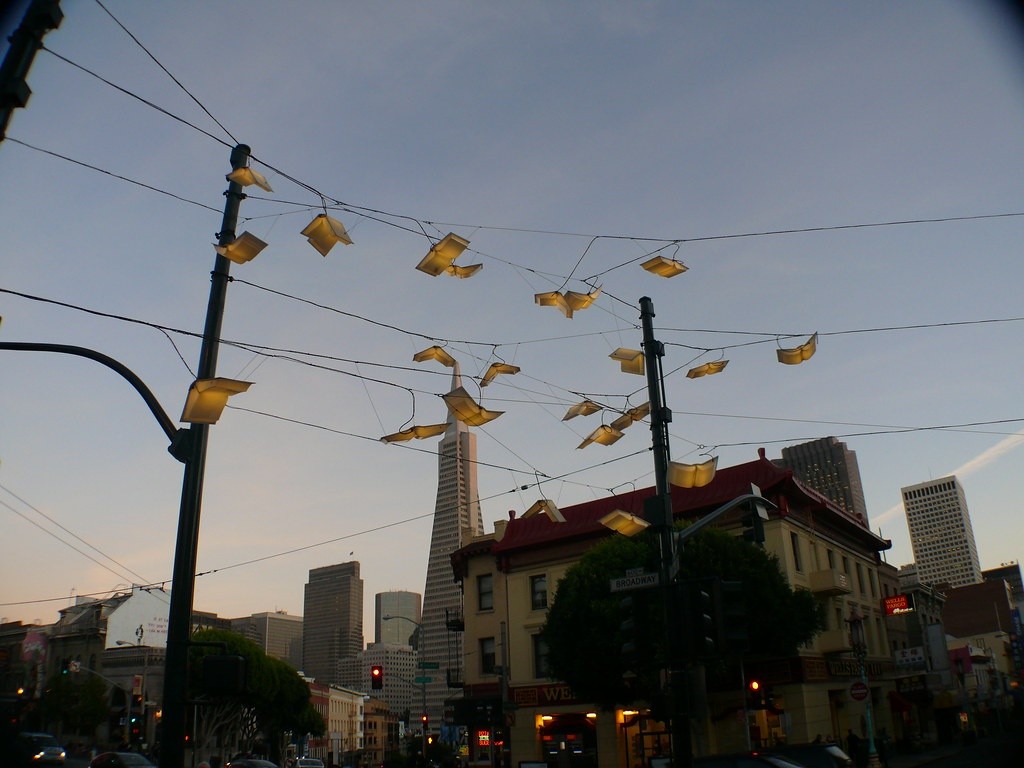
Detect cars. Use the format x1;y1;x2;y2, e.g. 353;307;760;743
227;758;279;768
291;759;325;768
664;751;808;768
12;731;66;768
87;751;157;768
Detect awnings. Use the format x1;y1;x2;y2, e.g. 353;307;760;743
888;690;911;713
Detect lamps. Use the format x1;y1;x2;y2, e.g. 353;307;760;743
669;444;719;487
300;195;354;257
226;155;274;193
211;229;268;266
595;482;650;539
179;377;256;425
520;471;567;522
377;218;729;453
776;332;817;365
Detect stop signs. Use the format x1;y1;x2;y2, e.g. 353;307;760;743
849;682;870;702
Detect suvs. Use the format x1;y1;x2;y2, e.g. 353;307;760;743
763;741;855;768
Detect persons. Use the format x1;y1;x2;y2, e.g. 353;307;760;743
116;735;133;752
847;729;859;745
812;734;835;744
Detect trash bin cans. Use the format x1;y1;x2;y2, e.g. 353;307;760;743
960;729;976;747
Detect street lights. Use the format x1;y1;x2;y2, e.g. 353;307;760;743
115;640;149;753
383;614;427;760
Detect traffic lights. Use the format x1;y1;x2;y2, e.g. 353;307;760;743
689;574;724;651
741;504;766;544
130;716;142;736
422;716;428;731
60;658;69;679
427;736;433;745
748;673;761;700
371;665;383;689
